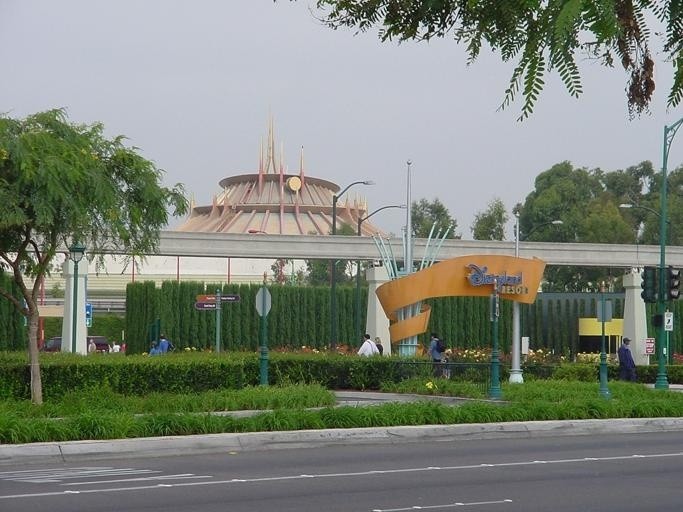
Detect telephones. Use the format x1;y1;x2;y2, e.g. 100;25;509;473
664;310;674;332
193;294;240;310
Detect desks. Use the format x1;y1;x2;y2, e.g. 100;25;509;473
355;201;407;349
65;235;87;356
327;177;379;350
247;227;297;285
618;203;677;390
507;208;565;384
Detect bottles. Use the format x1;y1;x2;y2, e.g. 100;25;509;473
623;337;631;341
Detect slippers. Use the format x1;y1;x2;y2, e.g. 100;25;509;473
433;340;446;353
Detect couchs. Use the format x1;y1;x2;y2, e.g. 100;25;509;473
638;263;657;304
667;262;681;301
652;314;665;328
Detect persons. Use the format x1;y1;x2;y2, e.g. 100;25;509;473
148;340;161;356
425;333;441;378
106;337;121;354
157;333;168;353
617;337;637;383
374;336;383;357
87;337;96;353
355;334;379;358
440;352;450;379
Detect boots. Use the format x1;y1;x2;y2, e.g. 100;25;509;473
39;334;111;356
